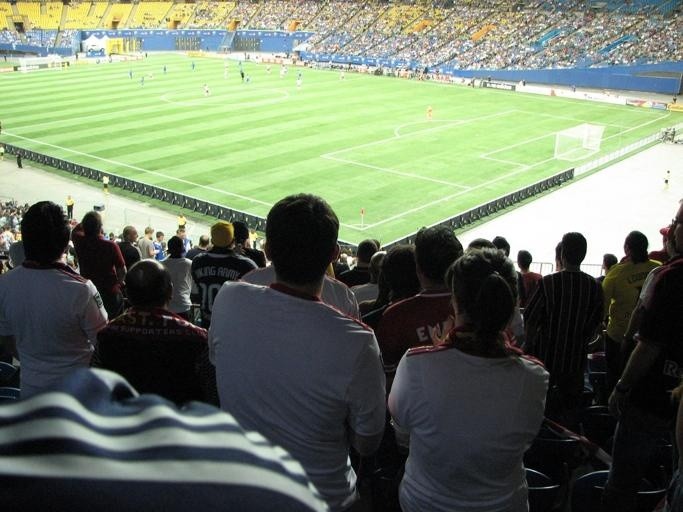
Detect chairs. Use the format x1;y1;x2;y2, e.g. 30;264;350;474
0;200;683;512
0;0;683;71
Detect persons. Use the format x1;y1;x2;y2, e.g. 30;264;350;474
664;170;670;182
0;143;4;161
1;191;682;511
425;106;432;121
659;127;677;142
0;0;682;108
15;149;23;168
102;174;109;194
662;179;670;196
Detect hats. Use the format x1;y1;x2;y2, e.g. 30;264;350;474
660;226;670;237
211;220;235;247
